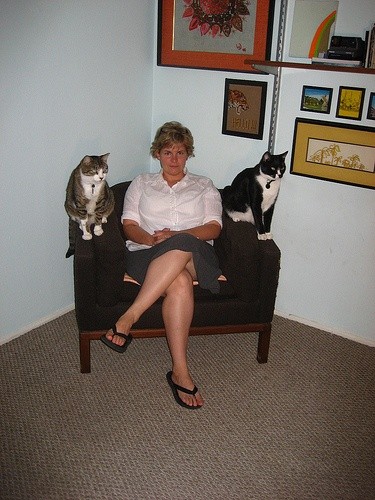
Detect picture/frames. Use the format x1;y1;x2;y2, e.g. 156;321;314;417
366;92;375;120
221;78;268;141
300;85;333;114
156;0;276;75
335;85;366;121
289;118;375;191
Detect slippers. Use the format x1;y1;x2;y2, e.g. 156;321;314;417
166;371;202;410
100;323;133;353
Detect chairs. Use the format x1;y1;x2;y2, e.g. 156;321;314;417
73;182;281;374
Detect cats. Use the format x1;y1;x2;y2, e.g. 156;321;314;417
64;152;116;241
221;150;289;240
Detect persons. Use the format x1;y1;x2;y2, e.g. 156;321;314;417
99;121;224;410
